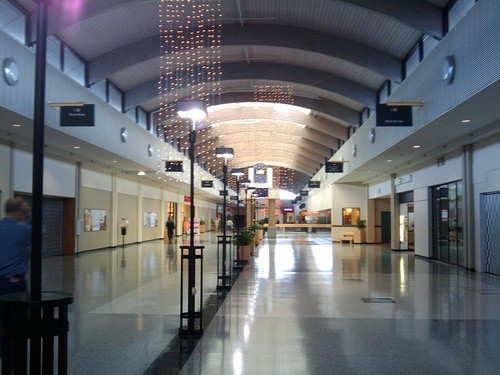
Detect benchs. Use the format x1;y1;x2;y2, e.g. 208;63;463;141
338;232;354;244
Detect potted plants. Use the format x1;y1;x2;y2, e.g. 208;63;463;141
200;220;206;233
354;220;366;243
233;221;263;261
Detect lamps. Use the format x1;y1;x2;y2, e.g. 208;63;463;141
216;148;234;165
231;169;244;181
176;101;207;130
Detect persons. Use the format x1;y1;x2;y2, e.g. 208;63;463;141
166;216;175;243
212;217;219;231
182;219;189;234
227;217;233;236
0;197;46;294
